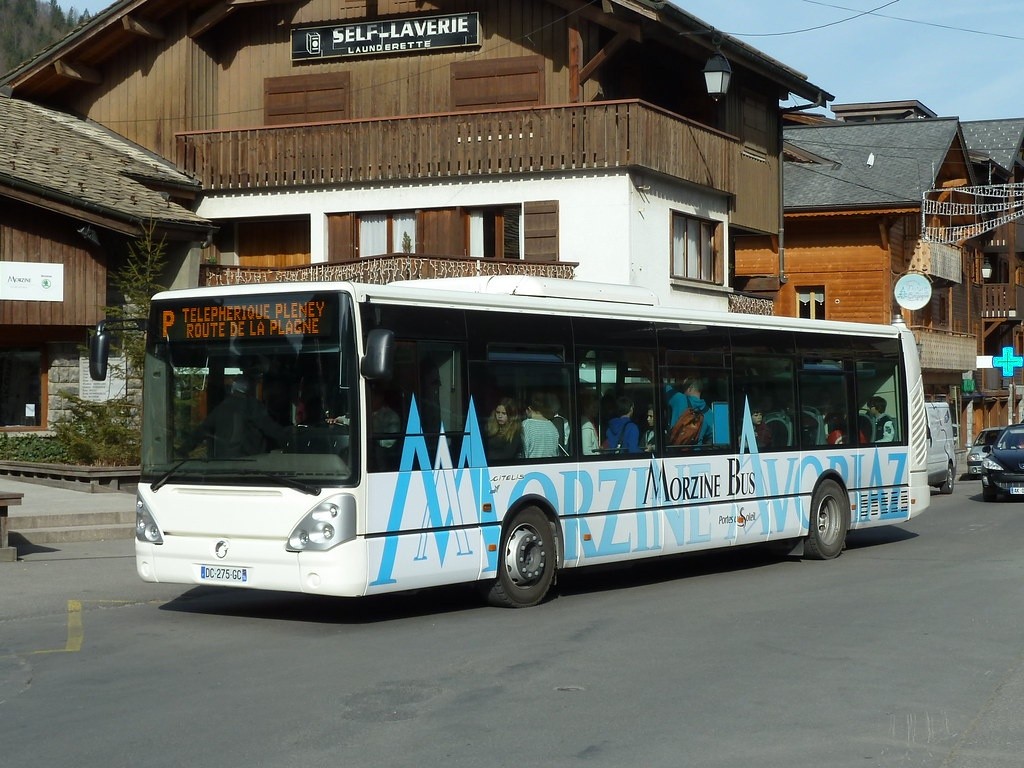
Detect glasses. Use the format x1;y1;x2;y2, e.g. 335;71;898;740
867;405;875;410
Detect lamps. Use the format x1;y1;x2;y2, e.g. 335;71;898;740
702;53;733;101
981;262;993;278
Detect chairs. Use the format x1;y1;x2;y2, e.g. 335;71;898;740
551;400;893;459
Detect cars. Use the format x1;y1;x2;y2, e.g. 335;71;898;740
964;426;1006;480
980;423;1024;501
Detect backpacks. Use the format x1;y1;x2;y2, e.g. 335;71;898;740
668;394;710;446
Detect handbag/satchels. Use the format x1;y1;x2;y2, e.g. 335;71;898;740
615;441;628;457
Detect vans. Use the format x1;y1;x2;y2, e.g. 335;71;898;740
925;402;957;494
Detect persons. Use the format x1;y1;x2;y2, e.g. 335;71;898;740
867;396;895;442
324;387;400;449
482;375;714;459
737;406;773;447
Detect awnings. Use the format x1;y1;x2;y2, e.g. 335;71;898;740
568;0;835;104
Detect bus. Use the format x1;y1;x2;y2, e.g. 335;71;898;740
87;272;933;612
